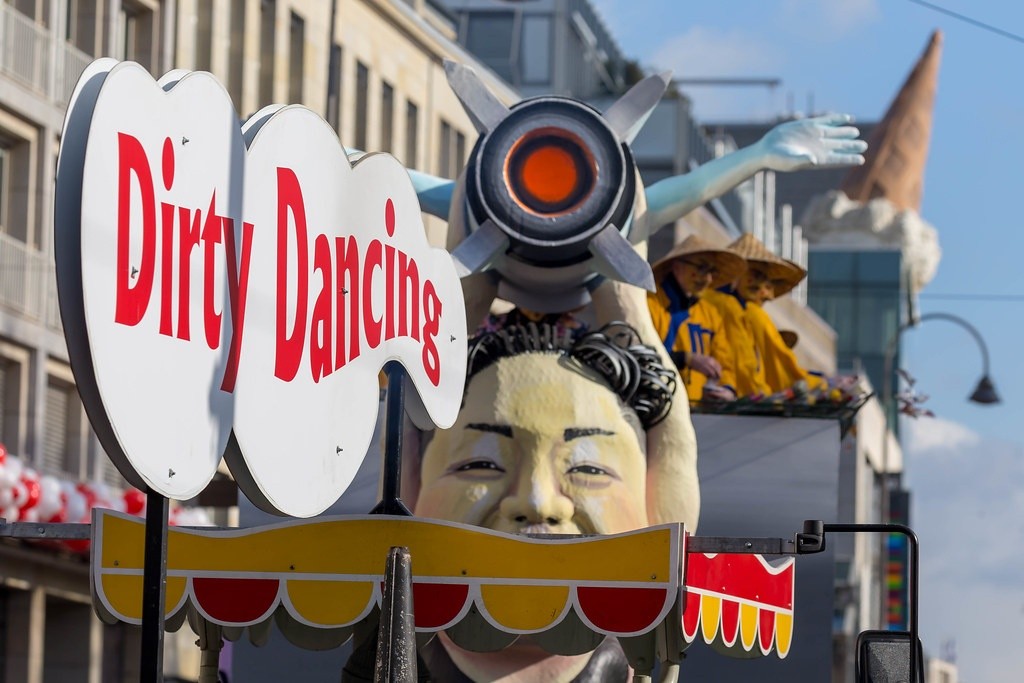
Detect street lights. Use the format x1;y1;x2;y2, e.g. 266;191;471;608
879;311;1004;629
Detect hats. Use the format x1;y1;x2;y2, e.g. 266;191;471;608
652;233;746;290
727;230;806;299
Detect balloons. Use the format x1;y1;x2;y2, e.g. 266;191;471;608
0;442;216;552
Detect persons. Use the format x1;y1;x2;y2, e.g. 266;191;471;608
648;232;842;404
405;113;868;246
341;162;700;683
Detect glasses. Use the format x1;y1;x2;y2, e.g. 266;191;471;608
678;257;720;279
